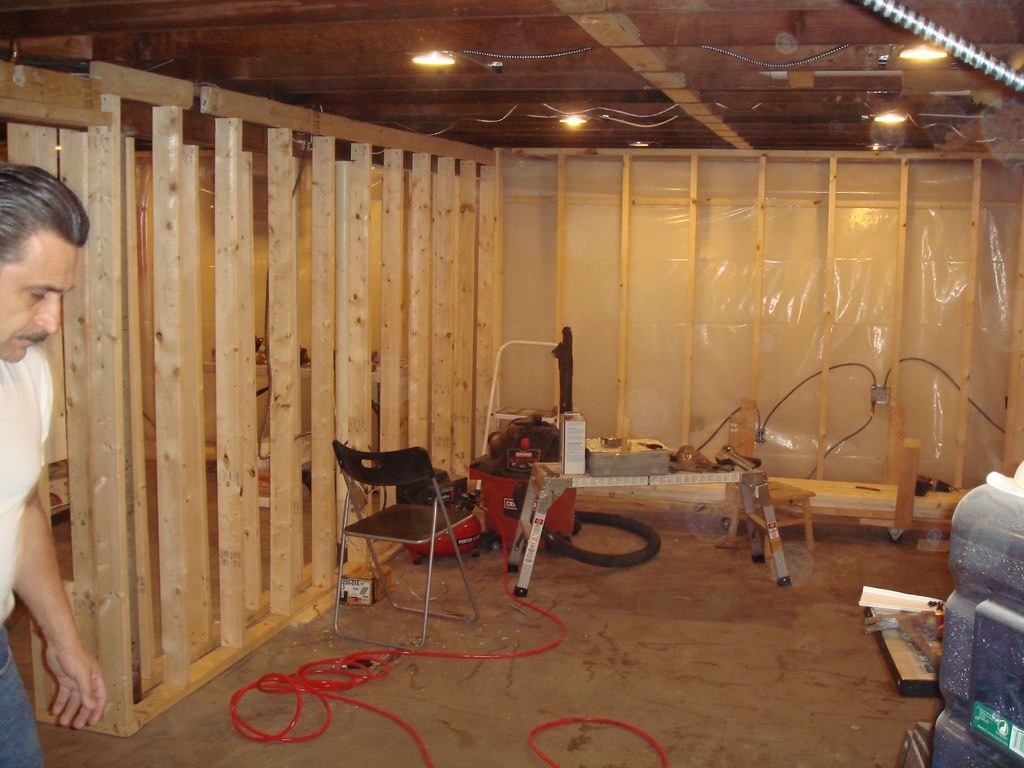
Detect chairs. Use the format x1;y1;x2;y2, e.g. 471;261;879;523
333;440;478;653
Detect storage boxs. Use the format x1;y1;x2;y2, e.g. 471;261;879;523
340;564;392;606
46;459;70;508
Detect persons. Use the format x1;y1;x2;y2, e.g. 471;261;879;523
0;162;106;768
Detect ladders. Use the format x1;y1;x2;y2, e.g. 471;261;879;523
475;339;561;498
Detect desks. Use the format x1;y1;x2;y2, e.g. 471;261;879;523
202;362;381;383
508;462;791;597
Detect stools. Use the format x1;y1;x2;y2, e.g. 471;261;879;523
723;480;816;560
